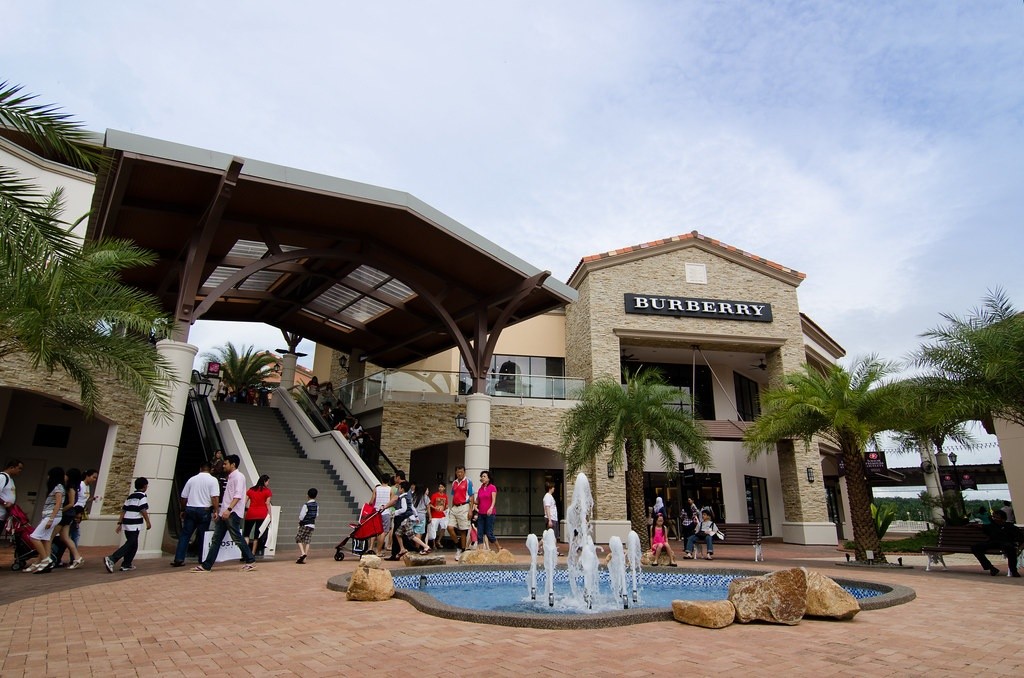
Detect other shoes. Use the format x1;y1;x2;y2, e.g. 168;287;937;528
1011;571;1021;577
989;567;999;576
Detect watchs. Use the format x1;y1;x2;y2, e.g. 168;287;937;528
3;501;7;506
228;507;232;511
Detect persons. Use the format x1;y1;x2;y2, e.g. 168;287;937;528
971;501;1024;578
648;496;724;568
38;471;84;570
367;465;568;564
304;376;374;457
103;477;152;573
170;448;317;574
50;468;100;569
0;461;23;536
21;467;65;574
216;376;273;406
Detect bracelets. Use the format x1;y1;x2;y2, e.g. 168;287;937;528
50;519;54;522
118;522;122;525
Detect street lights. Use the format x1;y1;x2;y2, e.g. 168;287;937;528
947;451;968;523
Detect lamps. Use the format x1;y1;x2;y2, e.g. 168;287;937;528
607;462;614;478
807;467;814;482
339;355;349;373
455;412;469;438
188;378;213;404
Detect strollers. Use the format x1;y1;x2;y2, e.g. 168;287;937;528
3;501;57;571
333;504;386;562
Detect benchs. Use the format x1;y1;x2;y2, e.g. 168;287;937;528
922;525;1024;577
693;523;763;561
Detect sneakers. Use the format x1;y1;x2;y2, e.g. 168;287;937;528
121;564;136;571
104;556;114;573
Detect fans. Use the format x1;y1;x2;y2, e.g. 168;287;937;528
748;359;767;370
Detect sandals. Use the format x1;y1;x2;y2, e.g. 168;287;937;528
65;557;84;570
169;538;727;571
22;558;41;572
32;557;53;574
